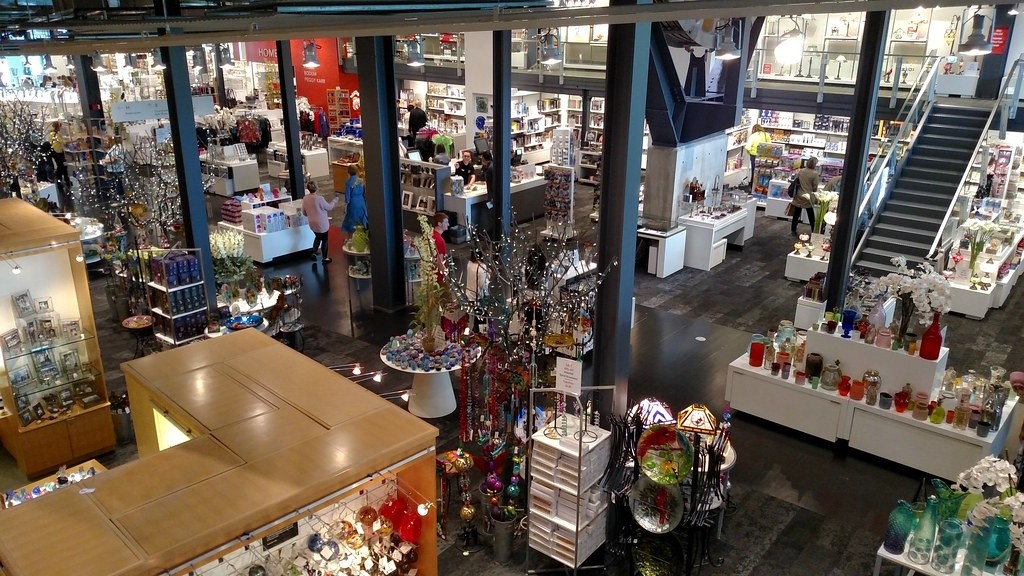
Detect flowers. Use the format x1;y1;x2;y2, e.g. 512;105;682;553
958;216;1002;266
802;190;838;232
949;453;1024;562
865;255;953;316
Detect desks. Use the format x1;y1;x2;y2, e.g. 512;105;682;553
201;158;260;196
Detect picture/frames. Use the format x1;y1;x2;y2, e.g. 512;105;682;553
1;289;82;389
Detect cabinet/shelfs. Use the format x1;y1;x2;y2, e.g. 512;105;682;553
266;85;907;357
218;220;320;264
875;527;1024;576
0;326;440;576
61;135;113;187
0;197;115;480
726;319;1023;489
528;414;612;570
940;197;1024;320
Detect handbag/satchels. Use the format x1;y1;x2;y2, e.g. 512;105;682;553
471;309;489;333
784;202;795;216
788;177;800;198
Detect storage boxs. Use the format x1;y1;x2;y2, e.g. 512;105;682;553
241;198;307;234
152;256;208;340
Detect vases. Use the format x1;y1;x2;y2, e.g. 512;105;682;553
747;299;1011;438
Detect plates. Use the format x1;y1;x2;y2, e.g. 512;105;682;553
204;274;304;341
630;391;739;576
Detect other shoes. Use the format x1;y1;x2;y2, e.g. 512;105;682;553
322;258;332;263
312;254;317;261
791;231;797;236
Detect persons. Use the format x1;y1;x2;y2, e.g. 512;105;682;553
1;73;124;215
302;181;339;264
790;157;820;237
341;165;368;241
396;100;496;332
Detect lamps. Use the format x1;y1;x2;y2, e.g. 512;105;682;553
303;39;322;69
247;475;437;575
327;364;419;403
537;31;566;66
0;45;234;85
715;19;742;62
49;210;105;241
404;34;425;68
957;5;994;56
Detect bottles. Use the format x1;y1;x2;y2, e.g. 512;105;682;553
747;292;1024;576
